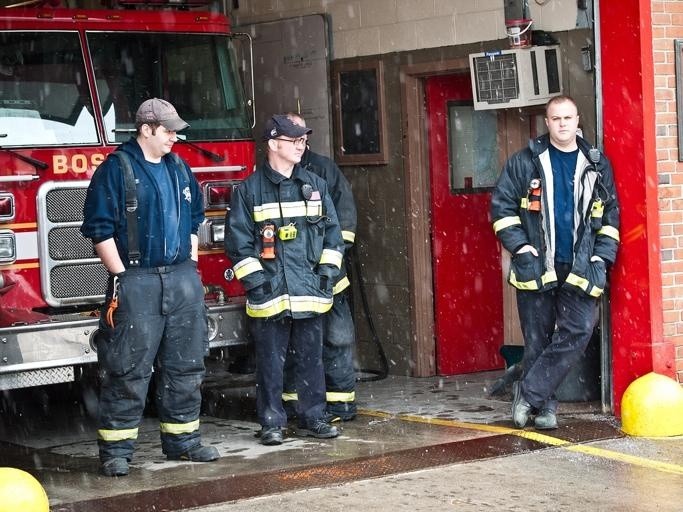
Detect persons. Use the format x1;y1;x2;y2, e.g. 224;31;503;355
489;94;620;431
78;95;220;477
225;114;347;446
281;113;359;423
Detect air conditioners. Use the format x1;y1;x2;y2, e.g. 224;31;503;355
469;42;564;112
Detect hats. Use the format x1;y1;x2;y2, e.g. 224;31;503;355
136;98;189;133
263;113;312;141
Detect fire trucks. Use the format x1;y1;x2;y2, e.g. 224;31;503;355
2;6;260;427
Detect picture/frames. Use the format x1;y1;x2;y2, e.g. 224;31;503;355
675;40;683;162
335;60;388;167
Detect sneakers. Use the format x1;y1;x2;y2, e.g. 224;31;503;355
534;408;560;431
261;424;284;445
511;380;533;429
295;411;357;438
167;444;221;462
103;457;130;475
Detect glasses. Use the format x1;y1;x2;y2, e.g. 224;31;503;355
275;138;305;147
